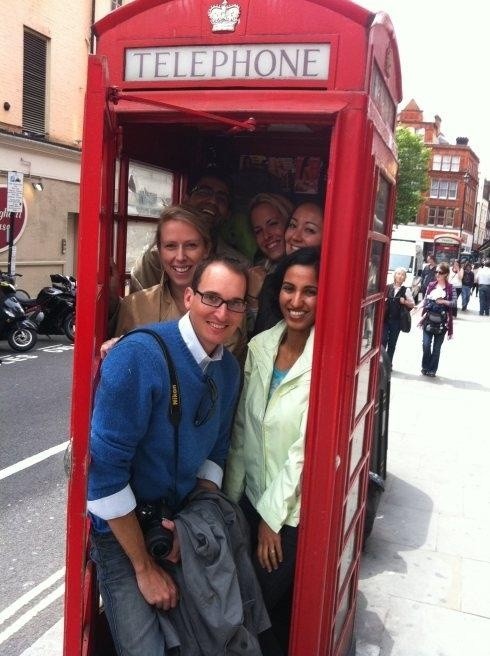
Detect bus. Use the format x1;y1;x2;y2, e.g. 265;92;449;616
431;233;464;265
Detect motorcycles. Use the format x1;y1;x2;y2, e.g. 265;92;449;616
0;269;75;352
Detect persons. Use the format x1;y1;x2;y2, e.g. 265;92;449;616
415;260;460;377
381;268;416;373
99;204;216;359
224;246;322;656
129;167;254;294
84;253;245;656
420;253;490;316
250;197;325;337
243;186;297;340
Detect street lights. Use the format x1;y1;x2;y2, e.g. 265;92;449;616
457;172;470;264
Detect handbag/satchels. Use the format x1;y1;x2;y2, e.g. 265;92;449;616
400;306;411;333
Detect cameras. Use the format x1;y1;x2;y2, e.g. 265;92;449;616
135;498;177;558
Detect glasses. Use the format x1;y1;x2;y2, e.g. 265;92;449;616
190;184;230;206
195;289;247;313
434;270;447;275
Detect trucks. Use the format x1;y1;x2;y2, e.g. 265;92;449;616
369;223;424;310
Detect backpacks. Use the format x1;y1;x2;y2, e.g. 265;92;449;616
424;300;448;336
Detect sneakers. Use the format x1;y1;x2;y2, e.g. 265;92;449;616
421;368;437;377
462;307;467;312
479;308;489;315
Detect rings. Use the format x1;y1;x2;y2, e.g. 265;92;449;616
270;550;276;554
